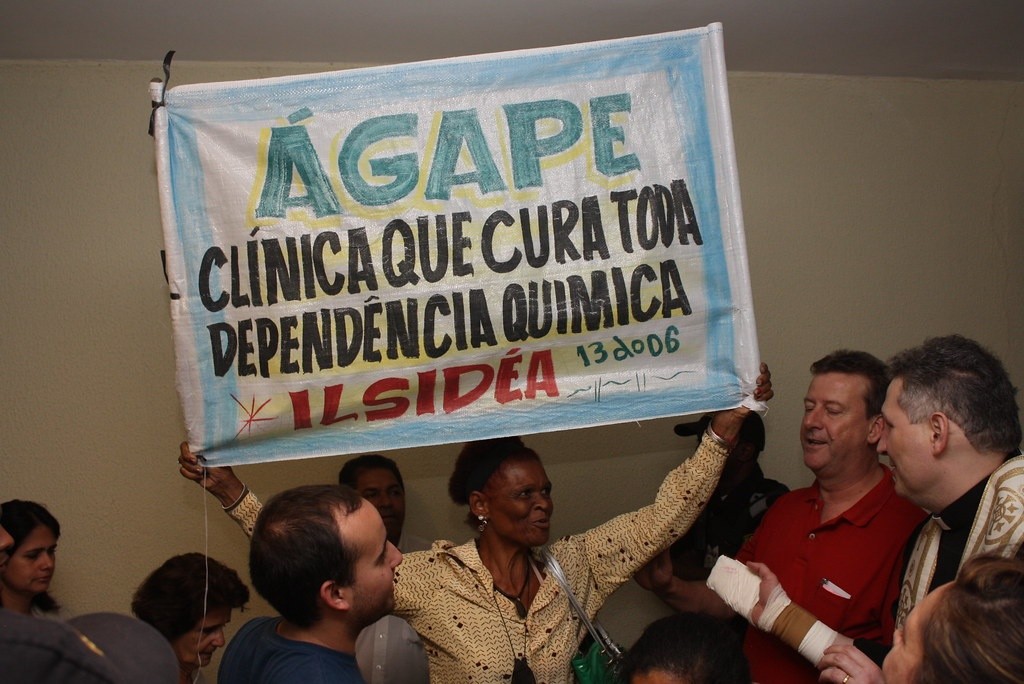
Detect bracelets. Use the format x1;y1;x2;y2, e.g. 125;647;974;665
708;420;737;448
220;482;247;511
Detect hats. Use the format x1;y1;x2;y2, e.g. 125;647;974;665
0;611;179;684
673;409;766;451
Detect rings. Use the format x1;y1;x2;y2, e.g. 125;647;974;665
842;675;849;683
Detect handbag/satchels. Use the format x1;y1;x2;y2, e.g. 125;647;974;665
540;545;629;684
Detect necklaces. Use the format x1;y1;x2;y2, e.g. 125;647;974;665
495;561;531;619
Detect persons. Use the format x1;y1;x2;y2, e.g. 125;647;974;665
631;406;790;583
131;551;249;684
218;482;404;684
177;361;773;684
338;452;430;684
622;606;750;684
631;350;930;684
706;334;1024;684
0;499;180;684
881;550;1024;684
